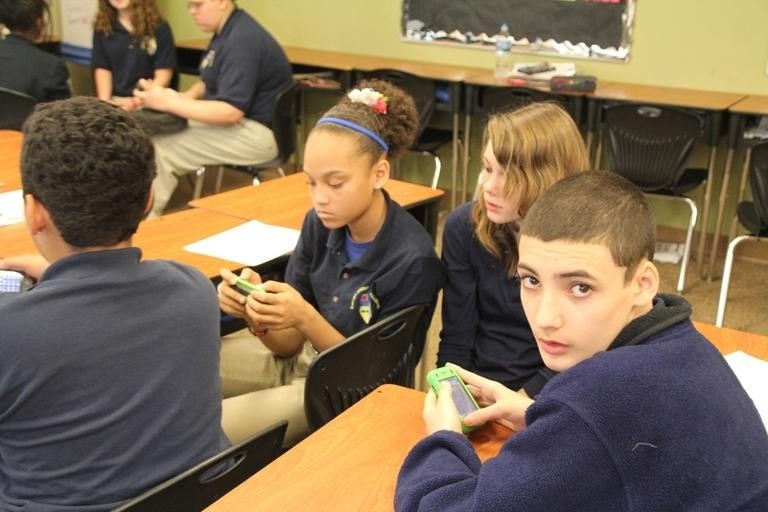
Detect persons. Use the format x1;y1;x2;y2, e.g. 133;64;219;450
0;95;237;512
219;75;448;453
393;166;768;512
0;0;72;102
436;98;591;402
90;0;179;110
131;0;294;221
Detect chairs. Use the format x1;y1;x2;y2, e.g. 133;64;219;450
0;36;765;511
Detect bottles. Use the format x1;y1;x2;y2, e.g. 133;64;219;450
492;21;513;80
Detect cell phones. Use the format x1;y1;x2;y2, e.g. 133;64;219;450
426;366;487;433
0;269;35;294
233;276;266;297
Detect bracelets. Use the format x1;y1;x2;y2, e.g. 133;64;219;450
247;323;269;337
